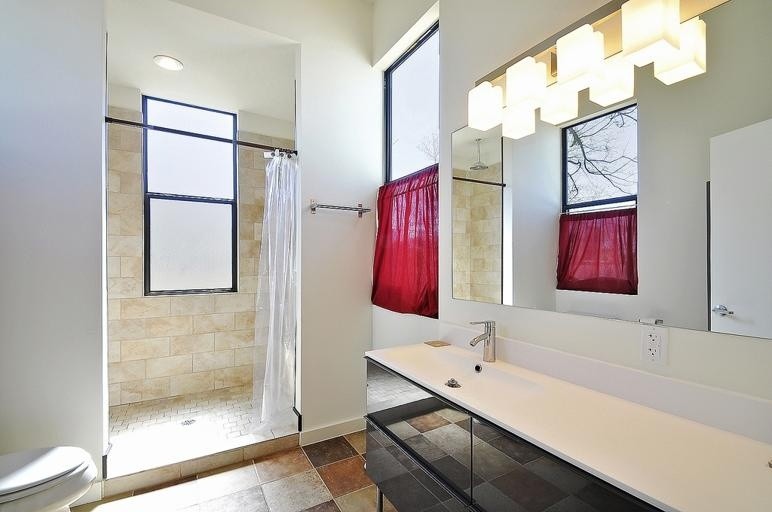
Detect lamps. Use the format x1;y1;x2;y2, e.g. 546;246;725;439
502;18;707;140
468;0;732;140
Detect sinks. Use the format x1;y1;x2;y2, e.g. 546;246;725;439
393;344;548;406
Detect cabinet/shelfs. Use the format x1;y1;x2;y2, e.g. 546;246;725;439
363;356;665;512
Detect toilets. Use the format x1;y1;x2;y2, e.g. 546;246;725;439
0;445;98;511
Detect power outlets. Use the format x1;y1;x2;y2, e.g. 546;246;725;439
641;327;669;366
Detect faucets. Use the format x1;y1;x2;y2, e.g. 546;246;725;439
469;320;494;364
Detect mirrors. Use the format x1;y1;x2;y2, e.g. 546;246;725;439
451;0;772;340
